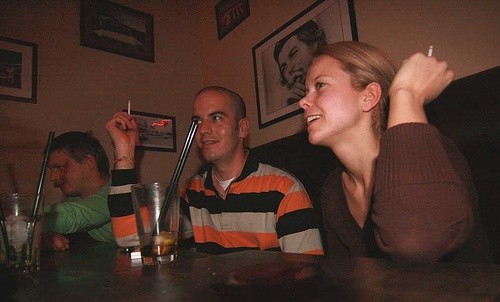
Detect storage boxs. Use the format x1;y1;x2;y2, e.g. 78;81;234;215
131;249;142;260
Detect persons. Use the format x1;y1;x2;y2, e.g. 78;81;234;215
298;41;481;263
36;131;114;251
105;86;324;255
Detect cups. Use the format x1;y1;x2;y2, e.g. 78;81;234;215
0;193;43;274
130;182;180;264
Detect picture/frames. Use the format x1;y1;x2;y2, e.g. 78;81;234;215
215;0;250;40
123;109;176;152
0;35;38;104
251;0;359;129
80;0;154;64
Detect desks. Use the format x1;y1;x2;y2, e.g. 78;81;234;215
1;233;500;302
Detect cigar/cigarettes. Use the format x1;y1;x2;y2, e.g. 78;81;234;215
128;100;131;115
428;46;433;56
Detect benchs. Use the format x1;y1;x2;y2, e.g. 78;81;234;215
199;65;500;264
